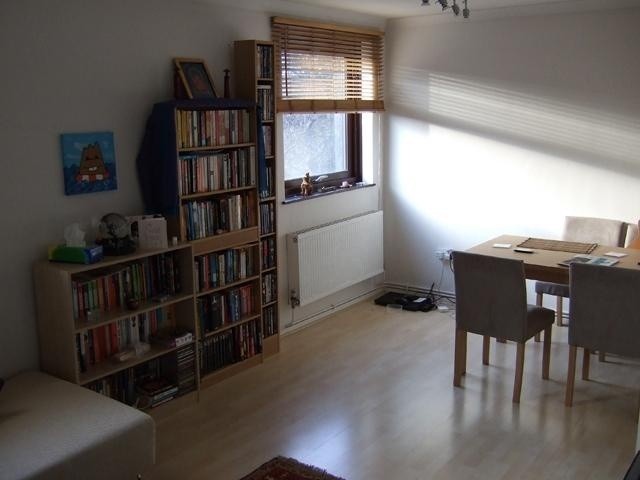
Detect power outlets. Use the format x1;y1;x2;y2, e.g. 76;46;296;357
432;249;456;263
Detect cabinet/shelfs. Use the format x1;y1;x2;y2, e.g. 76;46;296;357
156;103;263;389
34;245;198;407
235;38;278;350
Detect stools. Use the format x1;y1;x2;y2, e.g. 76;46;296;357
0;370;157;480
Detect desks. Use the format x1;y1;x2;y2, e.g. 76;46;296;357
461;229;639;280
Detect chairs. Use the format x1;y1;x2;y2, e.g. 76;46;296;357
534;215;630;364
564;260;639;407
452;253;556;408
622;221;640;251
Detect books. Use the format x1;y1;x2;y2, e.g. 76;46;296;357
602;250;628;259
492;242;513;249
557;253;621;270
258;44;275;121
72;255;195;410
177;109;279;376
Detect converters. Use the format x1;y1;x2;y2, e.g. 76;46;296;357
438;305;448;312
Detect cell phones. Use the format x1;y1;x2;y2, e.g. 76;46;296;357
514;248;535;253
494;244;511;248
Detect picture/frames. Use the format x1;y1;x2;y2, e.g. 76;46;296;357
176;58;220;100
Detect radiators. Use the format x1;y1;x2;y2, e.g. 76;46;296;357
286;210;384;309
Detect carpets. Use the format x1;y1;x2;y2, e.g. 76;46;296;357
240;455;344;480
